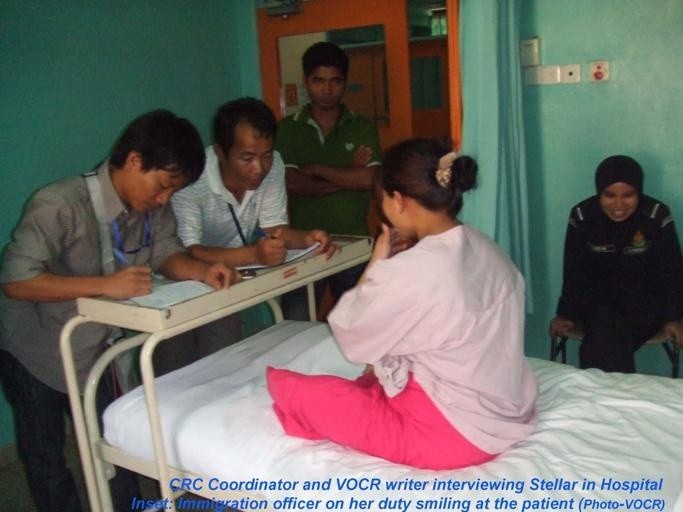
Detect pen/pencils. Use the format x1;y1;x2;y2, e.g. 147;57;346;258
262;233;280;239
112;247;133;268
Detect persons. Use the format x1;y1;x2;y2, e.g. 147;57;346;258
265;137;545;471
0;109;242;510
171;94;340;369
275;41;383;321
550;156;683;374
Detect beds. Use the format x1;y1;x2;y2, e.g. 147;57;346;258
51;304;682;511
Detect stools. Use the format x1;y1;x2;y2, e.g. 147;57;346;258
550;310;681;377
